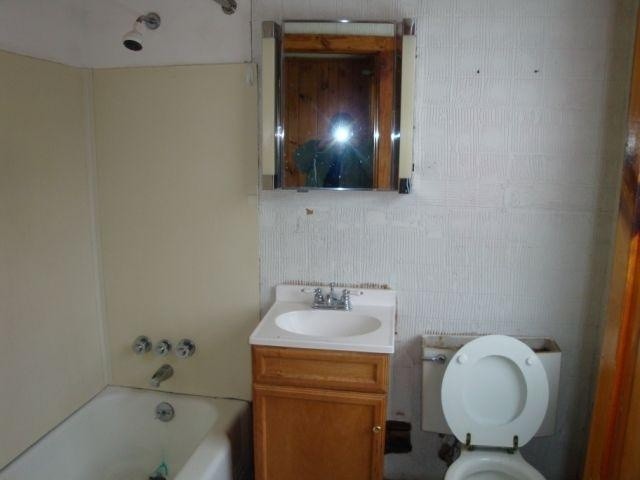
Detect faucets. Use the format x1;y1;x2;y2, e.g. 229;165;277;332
323;283;339;309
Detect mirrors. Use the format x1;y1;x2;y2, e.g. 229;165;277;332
259;16;416;193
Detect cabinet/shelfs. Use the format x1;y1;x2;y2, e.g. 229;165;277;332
250;346;388;480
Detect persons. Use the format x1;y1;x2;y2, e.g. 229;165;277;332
289;110;374;188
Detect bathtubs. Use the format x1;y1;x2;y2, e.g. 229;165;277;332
0;383;251;480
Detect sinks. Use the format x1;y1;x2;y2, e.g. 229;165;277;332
250;299;396;353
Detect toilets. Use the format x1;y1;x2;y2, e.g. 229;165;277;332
421;332;563;479
